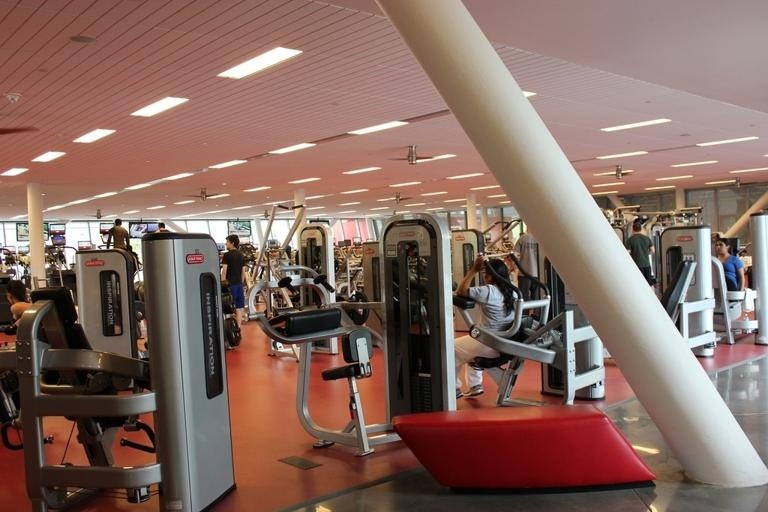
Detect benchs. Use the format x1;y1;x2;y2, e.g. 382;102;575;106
391;403;657;489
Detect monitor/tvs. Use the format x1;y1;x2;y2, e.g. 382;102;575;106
129;222;159;238
49;223;67;236
228;221;252;237
16;223;51;241
100;223;114;235
79;240;92;250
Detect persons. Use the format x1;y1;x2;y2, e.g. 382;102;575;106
106;219;131;252
454;250;516;400
220;234;246;327
154;222;171;235
625;223;656;291
512;224;538;320
0;277;37;336
714;237;748;300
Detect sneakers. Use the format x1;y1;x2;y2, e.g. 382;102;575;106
463;384;484;396
456;388;464;399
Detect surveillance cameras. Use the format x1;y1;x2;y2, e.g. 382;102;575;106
6;95;20;105
